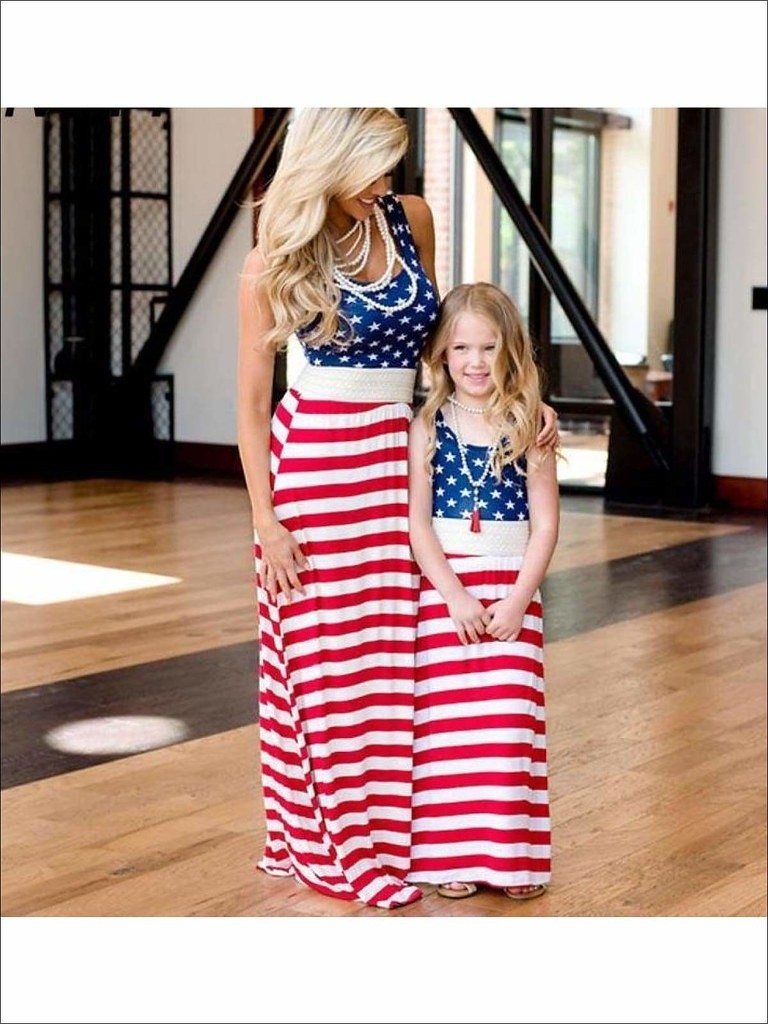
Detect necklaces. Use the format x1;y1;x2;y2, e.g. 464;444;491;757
331;204;418;311
446;391;511;533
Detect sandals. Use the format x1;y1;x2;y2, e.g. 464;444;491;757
436;882;477;899
504;883;548;900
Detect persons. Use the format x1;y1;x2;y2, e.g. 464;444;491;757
236;107;558;910
407;279;560;902
